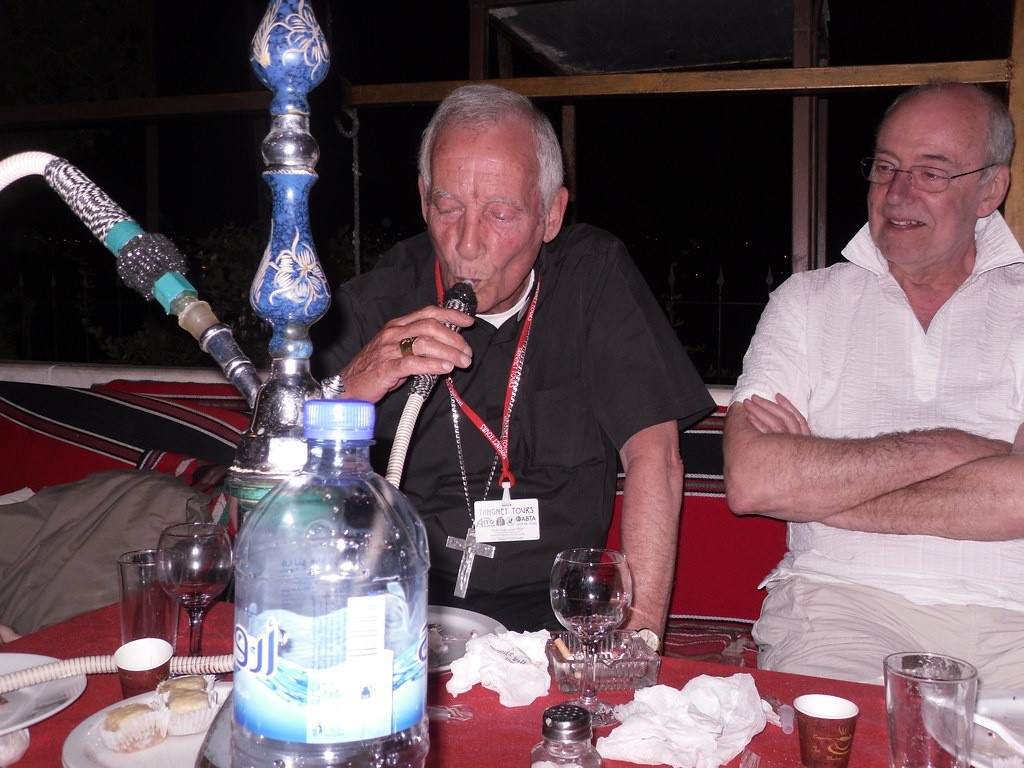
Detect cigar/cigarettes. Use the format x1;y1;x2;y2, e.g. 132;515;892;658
554;638;576;670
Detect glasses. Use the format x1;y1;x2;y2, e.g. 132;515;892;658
860;156;998;194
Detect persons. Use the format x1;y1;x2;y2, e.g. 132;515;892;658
309;85;719;658
723;79;1024;688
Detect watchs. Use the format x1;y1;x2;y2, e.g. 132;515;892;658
636;628;663;656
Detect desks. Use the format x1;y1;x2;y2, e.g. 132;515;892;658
0;590;960;768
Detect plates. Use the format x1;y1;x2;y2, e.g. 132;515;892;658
922;689;1024;768
61;681;232;768
0;652;87;736
426;605;509;676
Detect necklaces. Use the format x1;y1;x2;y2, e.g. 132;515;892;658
438;282;540;599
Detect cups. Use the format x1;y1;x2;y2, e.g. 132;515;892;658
114;637;173;698
883;652;979;768
793;694;859;768
116;549;184;654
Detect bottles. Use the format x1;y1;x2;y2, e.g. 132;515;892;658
230;399;432;768
530;705;602;768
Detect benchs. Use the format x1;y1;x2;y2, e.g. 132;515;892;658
0;359;789;672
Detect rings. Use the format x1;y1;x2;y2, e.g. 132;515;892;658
400;336;426;357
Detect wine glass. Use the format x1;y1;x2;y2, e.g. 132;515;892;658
550;548;634;727
156;523;233;657
